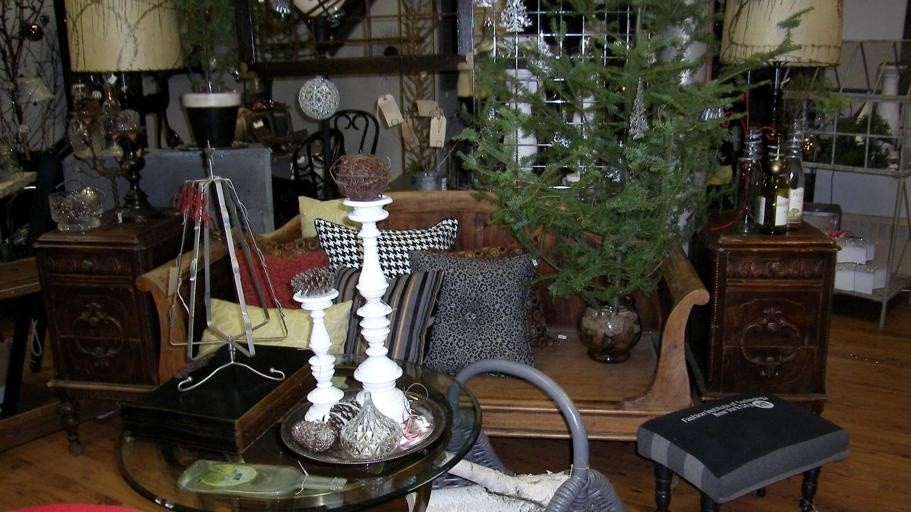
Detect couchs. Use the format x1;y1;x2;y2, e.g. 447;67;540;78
134;189;712;465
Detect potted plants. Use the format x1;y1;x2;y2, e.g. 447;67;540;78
175;0;242;148
451;2;815;364
399;1;447;190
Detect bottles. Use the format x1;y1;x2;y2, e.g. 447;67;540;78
737;117;807;236
340;392;401;460
177;457;348;498
67;81;141;159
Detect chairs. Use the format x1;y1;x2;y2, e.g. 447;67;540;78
292;109;380;201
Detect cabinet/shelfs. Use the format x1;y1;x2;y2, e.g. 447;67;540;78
773;37;911;328
684;220;843;415
34;204;206;460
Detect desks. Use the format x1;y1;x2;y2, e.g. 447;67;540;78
64;142;321;233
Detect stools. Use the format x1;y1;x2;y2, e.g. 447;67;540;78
636;391;849;511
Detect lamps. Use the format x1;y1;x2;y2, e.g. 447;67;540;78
720;0;846;161
64;0;184;226
